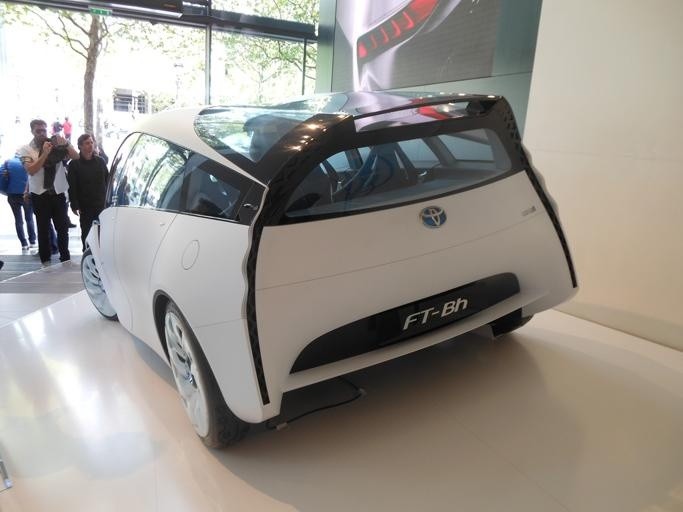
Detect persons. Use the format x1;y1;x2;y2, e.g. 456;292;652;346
109;153;154;209
0;116;111;271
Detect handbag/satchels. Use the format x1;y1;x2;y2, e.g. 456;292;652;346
2;170;9;189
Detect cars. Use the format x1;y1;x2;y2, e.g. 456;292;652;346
78;87;581;452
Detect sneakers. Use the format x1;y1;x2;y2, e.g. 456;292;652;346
68;224;76;228
22;243;36;250
41;262;51;268
